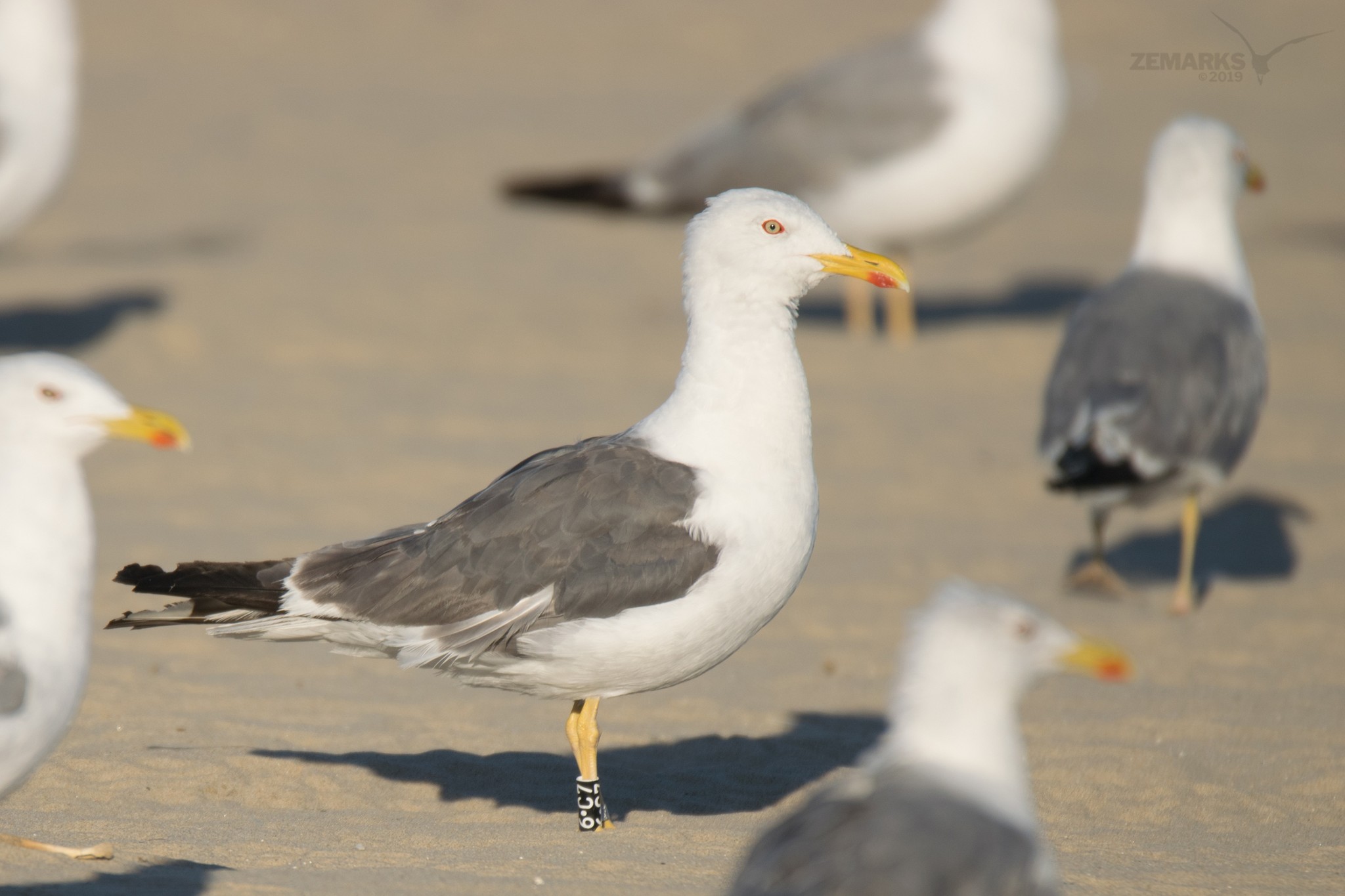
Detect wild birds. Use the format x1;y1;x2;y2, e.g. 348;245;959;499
103;182;918;833
2;348;201;863
480;1;1075;358
715;573;1146;896
1029;105;1269;618
2;0;86;259
